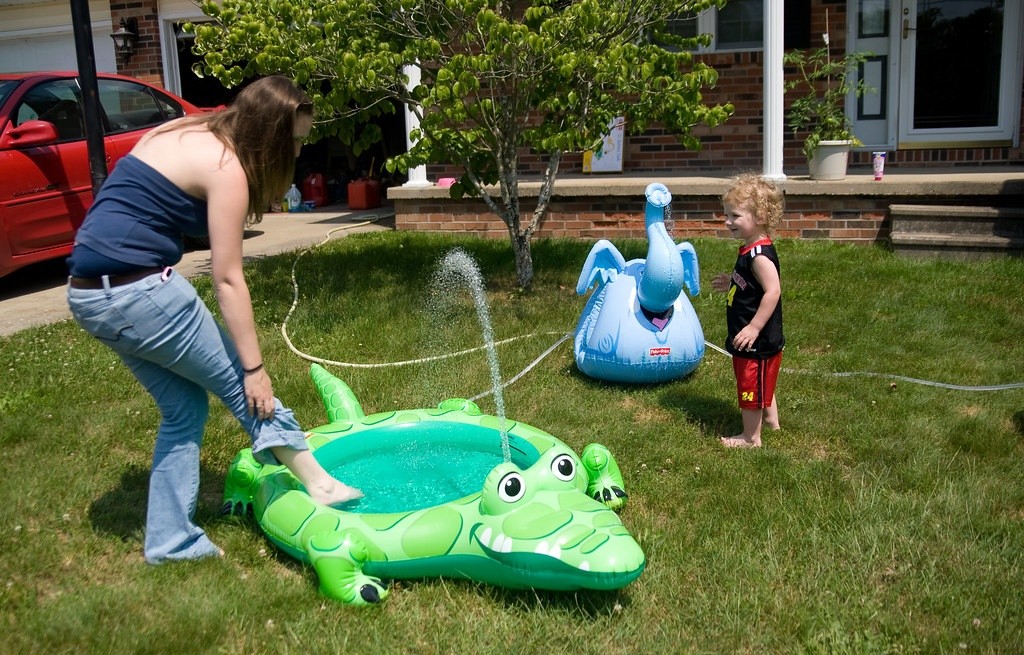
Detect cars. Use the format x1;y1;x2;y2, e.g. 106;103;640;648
0;69;229;278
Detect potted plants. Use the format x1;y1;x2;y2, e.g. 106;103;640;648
782;46;879;180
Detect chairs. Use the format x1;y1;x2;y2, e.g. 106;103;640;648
37;100;85;140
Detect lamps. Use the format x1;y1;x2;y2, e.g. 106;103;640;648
109;17;139;66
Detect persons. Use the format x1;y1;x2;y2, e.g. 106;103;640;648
67;75;364;565
710;172;785;448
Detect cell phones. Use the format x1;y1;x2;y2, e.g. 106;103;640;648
161;267;173;281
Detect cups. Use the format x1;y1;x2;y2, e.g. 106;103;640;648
873;152;886;181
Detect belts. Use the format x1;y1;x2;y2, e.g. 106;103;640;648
71;267;172;290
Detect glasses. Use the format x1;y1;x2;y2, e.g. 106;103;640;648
295;136;308;146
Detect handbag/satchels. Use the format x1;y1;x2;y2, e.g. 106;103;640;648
304;173;324;205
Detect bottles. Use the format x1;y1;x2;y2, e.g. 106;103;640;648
303;173;325;207
287;184;301;212
283;196;288;212
347;177;379;209
